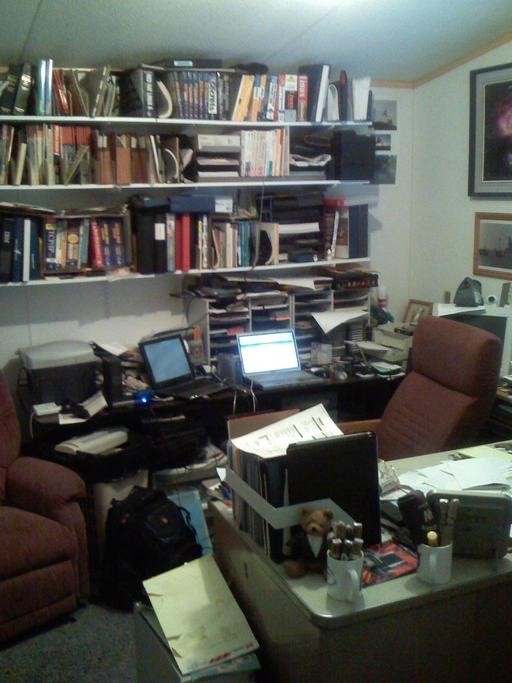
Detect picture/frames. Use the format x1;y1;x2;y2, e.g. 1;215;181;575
469;211;511;279
465;60;511;199
403;298;434;325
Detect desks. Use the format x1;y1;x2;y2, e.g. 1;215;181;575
14;356;376;428
203;436;511;681
488;382;511;433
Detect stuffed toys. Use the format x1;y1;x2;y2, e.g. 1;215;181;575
282;508;334;577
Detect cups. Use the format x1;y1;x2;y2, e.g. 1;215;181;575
417;541;454;586
327;546;364;603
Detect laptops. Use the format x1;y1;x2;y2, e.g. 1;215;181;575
236;329;323;389
138;334;233;400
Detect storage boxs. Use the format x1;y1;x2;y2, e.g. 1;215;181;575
16;334;104;404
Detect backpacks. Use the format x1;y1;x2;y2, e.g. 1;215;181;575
100;484;204;611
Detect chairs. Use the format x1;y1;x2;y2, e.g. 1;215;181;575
332;310;506;461
0;369;96;650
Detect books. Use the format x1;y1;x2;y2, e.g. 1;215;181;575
229;406;381;553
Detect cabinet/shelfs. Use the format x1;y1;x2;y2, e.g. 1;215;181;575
1;110;378;290
185;282;373;369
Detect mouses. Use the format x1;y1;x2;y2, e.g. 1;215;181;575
315;371;329;377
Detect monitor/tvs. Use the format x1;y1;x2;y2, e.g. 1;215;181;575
436;306;512;379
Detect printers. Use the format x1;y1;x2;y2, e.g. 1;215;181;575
356;324;414;371
